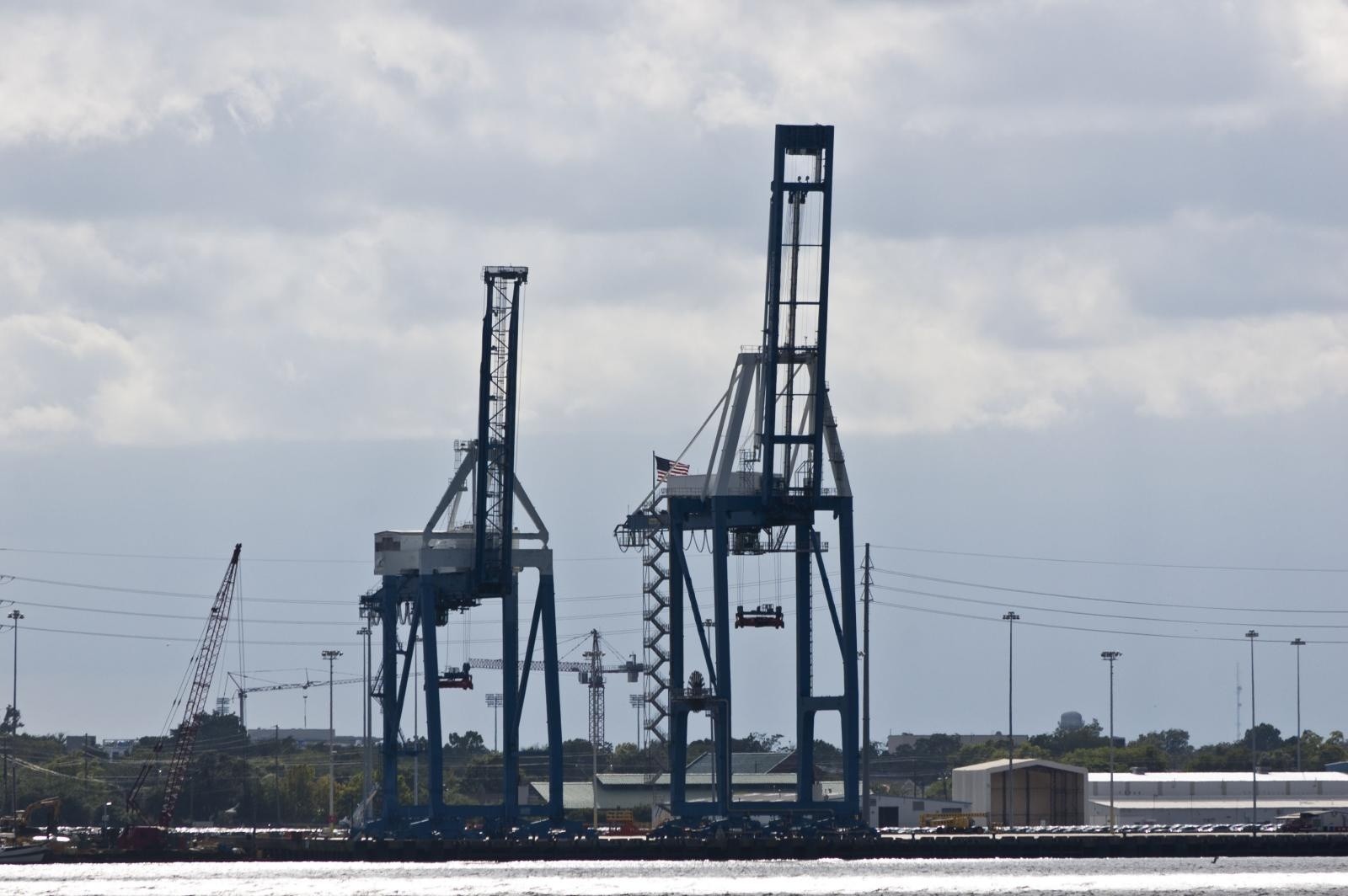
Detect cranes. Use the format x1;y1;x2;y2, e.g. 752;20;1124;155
469;629;648;760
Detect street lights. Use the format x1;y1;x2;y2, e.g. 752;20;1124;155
1290;637;1306;773
321;649;344;835
1003;612;1020;828
1101;651;1123;836
1245;629;1259;840
6;609;25;845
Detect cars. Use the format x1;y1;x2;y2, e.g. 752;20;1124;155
983;824;1284;833
875;826;940;834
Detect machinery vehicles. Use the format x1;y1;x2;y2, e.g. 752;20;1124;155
920;812;992;835
112;540;244;857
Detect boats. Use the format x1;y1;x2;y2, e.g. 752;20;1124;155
0;838;54;864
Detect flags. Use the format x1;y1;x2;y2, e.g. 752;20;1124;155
655;456;690;482
